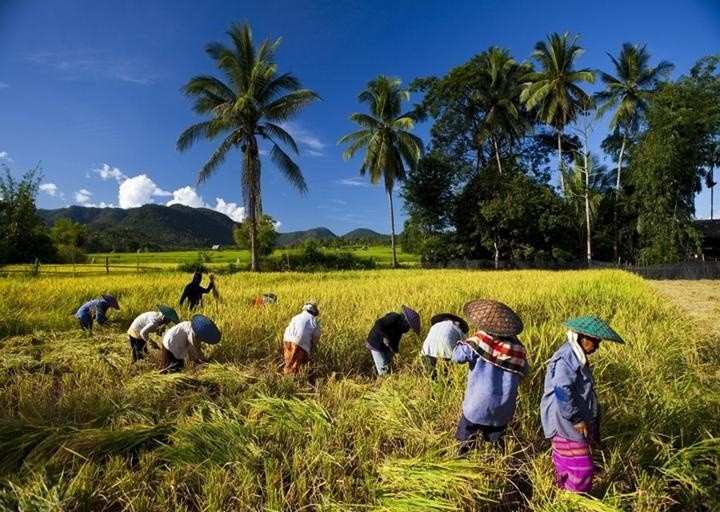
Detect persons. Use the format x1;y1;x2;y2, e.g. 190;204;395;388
451;299;527;463
127;303;180;364
159;314;222;375
539;315;626;497
177;271;213;313
252;293;277;305
421;313;468;401
282;303;321;393
75;294;121;339
364;304;421;382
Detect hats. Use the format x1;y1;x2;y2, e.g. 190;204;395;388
156;303;180;324
191;314;221;344
431;313;469;333
560;315;625;346
463;299;523;337
101;294;120;310
401;304;420;337
